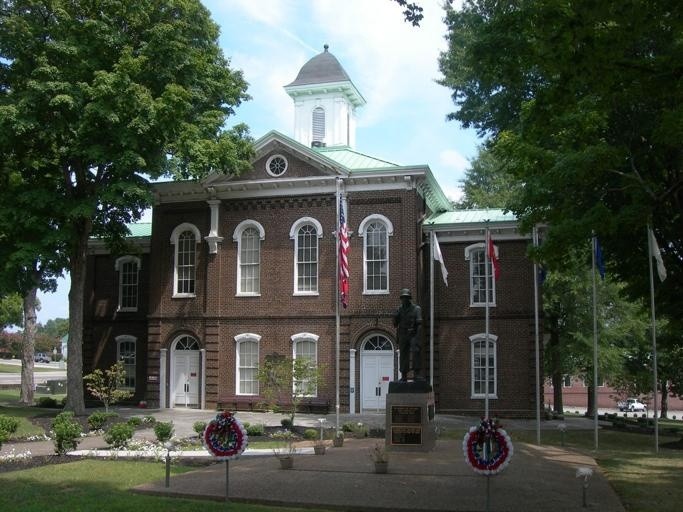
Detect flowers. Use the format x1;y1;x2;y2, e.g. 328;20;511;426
202;417;248;461
479;416;502;441
463;424;513;476
216;409;235;424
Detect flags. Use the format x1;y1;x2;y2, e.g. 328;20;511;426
433;232;450;287
535;232;547;286
487;229;500;281
651;229;667;283
594;234;605;281
339;194;350;308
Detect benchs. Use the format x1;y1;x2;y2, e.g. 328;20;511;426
275;397;331;414
217;396;270;412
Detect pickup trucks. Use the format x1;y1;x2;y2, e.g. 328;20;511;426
33;352;50;363
618;396;647;412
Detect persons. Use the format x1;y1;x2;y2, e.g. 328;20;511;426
392;288;423;382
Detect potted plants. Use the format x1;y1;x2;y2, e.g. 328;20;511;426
342;421;369;439
273;441;299;469
332;431;344;447
311;437;332;455
367;442;392;473
